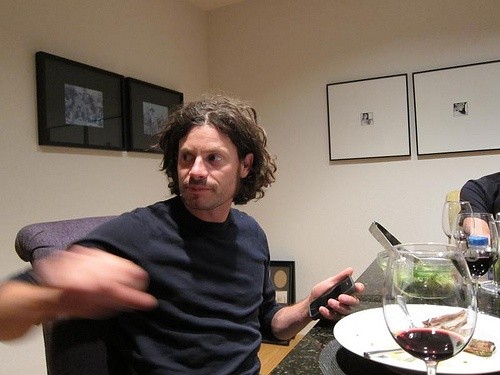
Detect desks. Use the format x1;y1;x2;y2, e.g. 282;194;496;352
270;255;500;375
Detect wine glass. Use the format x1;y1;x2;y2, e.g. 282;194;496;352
480;212;500;296
382;241;478;375
450;212;500;315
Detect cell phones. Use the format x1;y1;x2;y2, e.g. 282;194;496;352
309;274;356;320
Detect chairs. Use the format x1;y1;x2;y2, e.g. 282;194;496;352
14;215;121;375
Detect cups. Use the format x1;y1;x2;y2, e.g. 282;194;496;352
442;201;475;248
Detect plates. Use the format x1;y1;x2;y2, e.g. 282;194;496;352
333;305;500;375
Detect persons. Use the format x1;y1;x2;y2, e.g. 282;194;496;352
459;172;500;254
362;113;373;125
32;98;364;375
454;102;468;118
0;279;77;340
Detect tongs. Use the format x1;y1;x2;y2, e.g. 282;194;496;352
369;221;424;265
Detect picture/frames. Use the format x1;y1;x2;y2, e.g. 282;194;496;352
35;51;124;150
125;76;184;153
326;74;411;161
412;60;500;156
